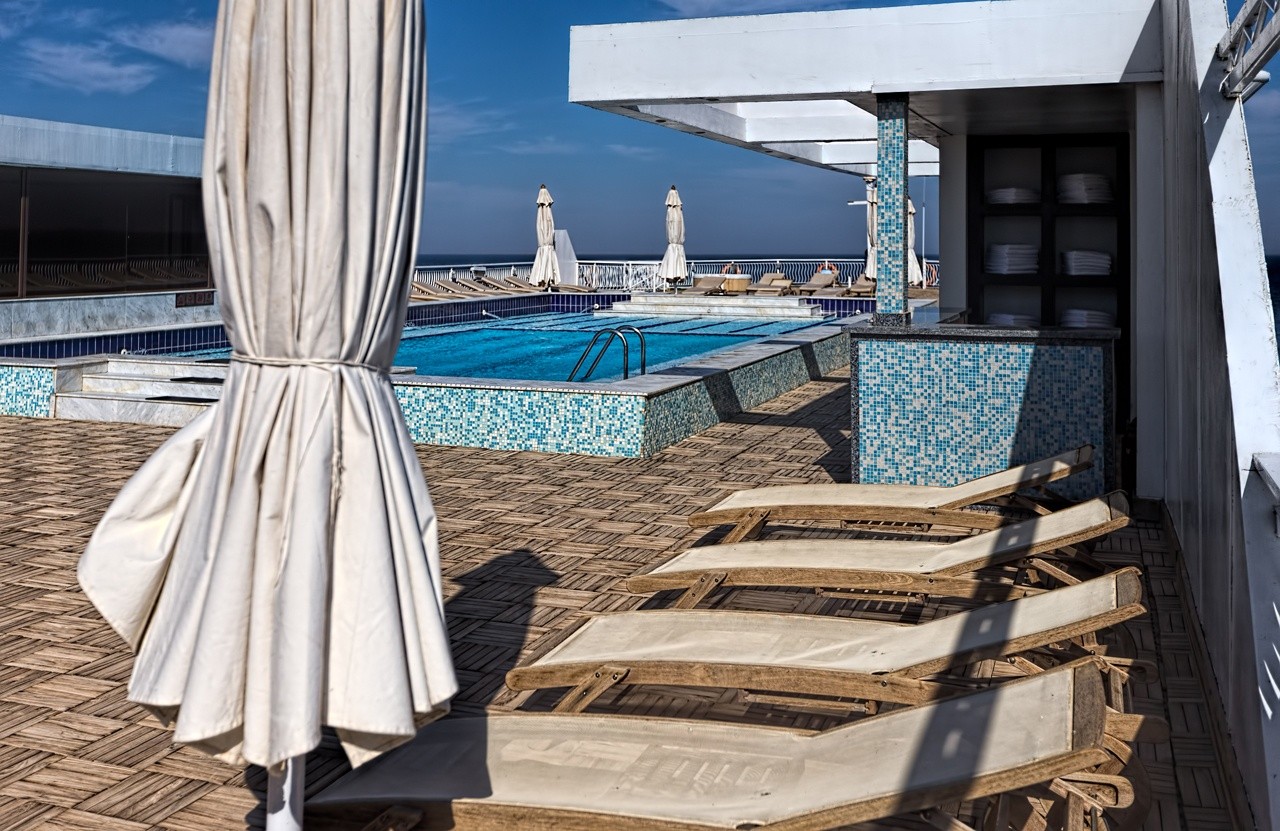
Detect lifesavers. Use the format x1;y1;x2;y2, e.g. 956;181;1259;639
721;263;741;274
926;264;936;286
817;263;837;272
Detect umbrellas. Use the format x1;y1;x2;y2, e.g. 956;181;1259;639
528;184;561;293
907;195;923;286
864;183;877;297
74;0;458;831
657;185;689;294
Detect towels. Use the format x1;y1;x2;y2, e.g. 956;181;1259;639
1061;246;1112;275
988;187;1039;203
988;312;1039;327
1060;308;1114;328
983;243;1041;274
1057;173;1114;203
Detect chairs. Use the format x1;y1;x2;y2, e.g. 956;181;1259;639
409;277;597;303
0;267;208;294
746;271;876;298
683;275;727;296
307;446;1171;831
